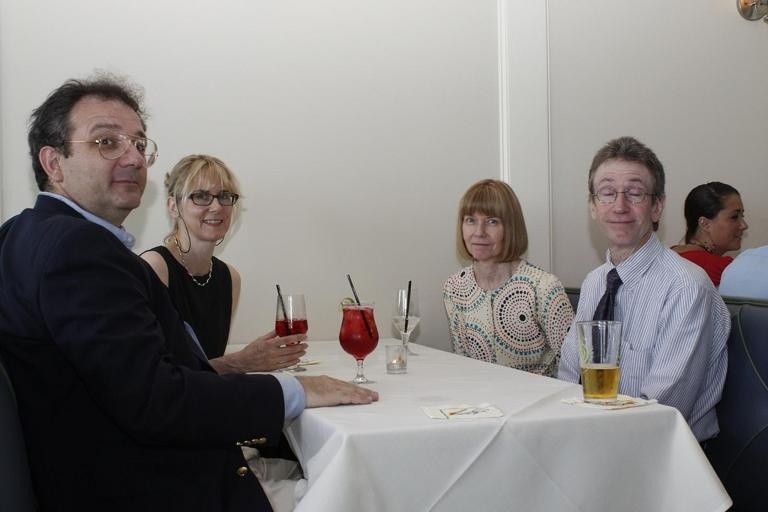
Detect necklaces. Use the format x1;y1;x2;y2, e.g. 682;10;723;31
174;237;212;286
689;243;709;251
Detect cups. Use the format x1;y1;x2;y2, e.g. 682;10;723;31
576;321;622;404
385;345;408;375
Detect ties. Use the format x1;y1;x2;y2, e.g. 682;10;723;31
591;268;622;391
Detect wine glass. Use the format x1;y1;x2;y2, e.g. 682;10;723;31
337;299;378;386
274;294;308;374
392;290;422;357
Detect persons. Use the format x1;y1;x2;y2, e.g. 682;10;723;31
444;178;575;377
138;154;308;374
718;246;767;298
557;135;732;460
671;182;748;285
1;73;380;512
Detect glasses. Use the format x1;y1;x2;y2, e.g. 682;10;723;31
60;133;160;170
179;191;241;207
590;185;658;204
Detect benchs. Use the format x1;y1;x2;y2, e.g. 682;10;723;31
701;295;768;511
